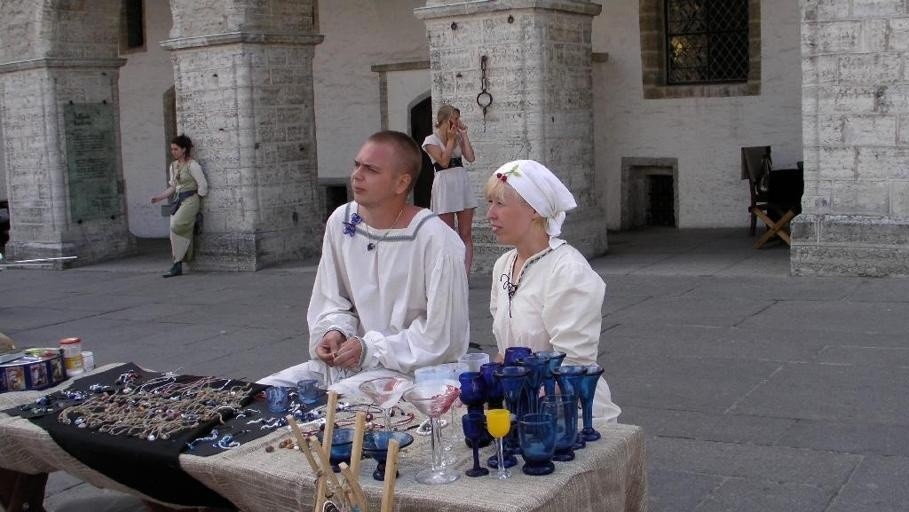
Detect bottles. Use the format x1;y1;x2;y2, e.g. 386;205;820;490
60;336;83;377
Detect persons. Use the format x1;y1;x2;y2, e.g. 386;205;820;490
251;130;471;396
420;103;480;290
482;158;622;422
149;134;209;278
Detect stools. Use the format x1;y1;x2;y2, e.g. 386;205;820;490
747;204;800;249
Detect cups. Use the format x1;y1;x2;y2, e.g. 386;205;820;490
267;375;320;412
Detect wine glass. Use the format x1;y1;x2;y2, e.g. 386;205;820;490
360;361;456;485
457;340;604;480
323;428;411;479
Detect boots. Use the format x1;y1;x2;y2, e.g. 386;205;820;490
195;213;203;234
162;262;182;277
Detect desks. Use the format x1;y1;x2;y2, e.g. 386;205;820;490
762;168;804;243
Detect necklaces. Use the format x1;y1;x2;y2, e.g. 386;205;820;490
362;203;406;250
56;366;251;439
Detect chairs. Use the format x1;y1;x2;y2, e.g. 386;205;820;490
741;145;773;235
